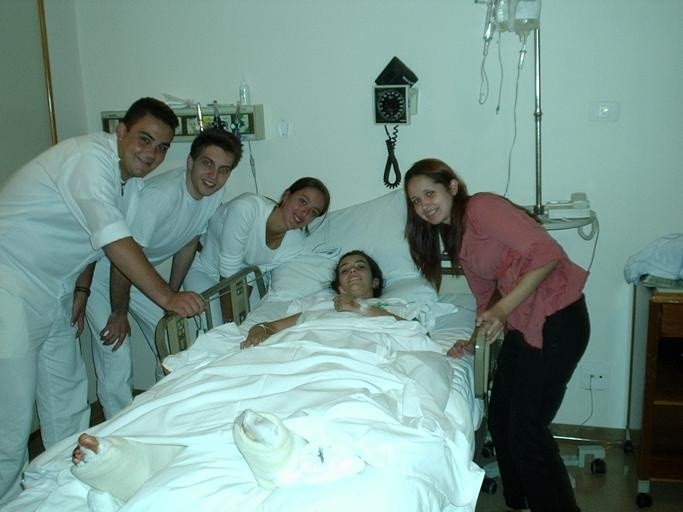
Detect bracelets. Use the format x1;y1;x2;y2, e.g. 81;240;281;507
75;285;92;298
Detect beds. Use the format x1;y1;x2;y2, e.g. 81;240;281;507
0;187;504;511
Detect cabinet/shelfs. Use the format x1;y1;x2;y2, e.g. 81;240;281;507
636;300;683;512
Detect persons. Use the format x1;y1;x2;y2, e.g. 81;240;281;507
84;130;243;420
183;176;330;342
70;251;431;503
403;159;590;512
0;96;205;502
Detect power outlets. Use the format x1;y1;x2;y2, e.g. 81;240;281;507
580;365;609;390
591;100;620;121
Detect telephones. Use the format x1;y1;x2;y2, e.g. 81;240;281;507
547;192;592;220
372;85;419;126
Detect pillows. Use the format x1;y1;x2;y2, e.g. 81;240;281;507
267;187;439;304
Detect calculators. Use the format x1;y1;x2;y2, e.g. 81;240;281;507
640;274;678;288
